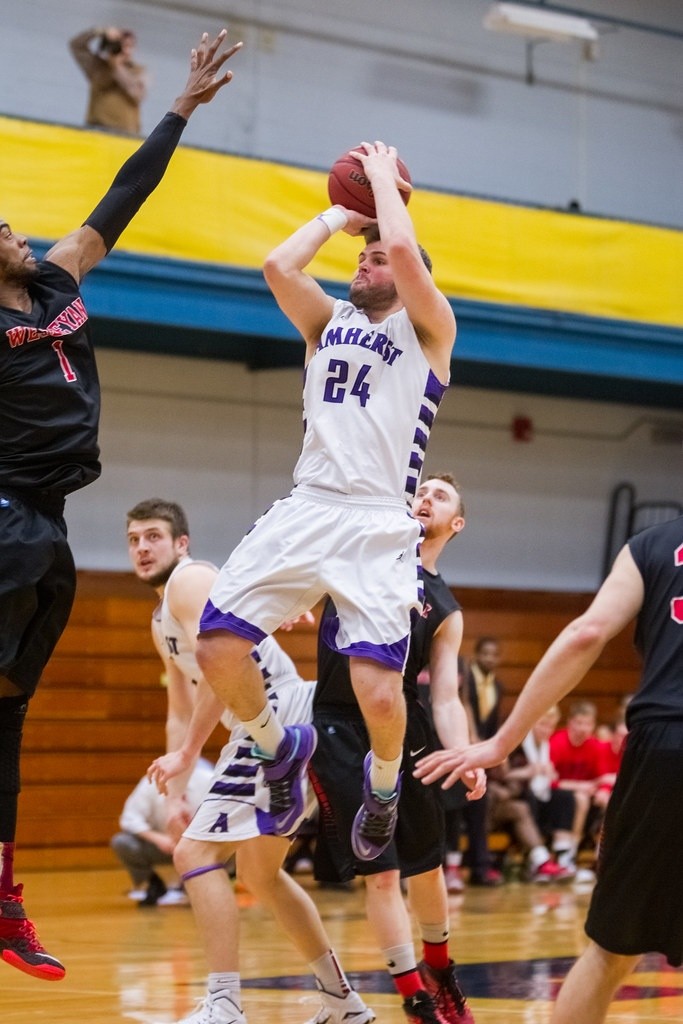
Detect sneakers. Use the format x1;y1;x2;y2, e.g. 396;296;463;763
418;958;475;1024
262;724;318;837
0;883;66;981
351;750;404;860
169;989;246;1024
295;989;375;1024
400;990;450;1024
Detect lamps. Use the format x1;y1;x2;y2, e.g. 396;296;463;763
482;4;598;42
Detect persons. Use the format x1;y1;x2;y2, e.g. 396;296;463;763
196;139;460;861
446;638;635;892
111;758;216;907
413;512;683;1024
0;28;244;981
69;27;146;136
279;473;486;1023
128;498;378;1024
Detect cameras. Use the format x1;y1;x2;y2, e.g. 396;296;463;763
110;40;121;53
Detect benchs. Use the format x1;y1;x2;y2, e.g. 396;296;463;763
14;568;646;874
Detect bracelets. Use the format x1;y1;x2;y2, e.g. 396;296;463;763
318;208;347;235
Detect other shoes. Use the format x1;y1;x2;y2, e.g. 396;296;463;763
130;888;189;904
401;848;597;893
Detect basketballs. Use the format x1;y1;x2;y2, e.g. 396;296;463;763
327;147;412;231
